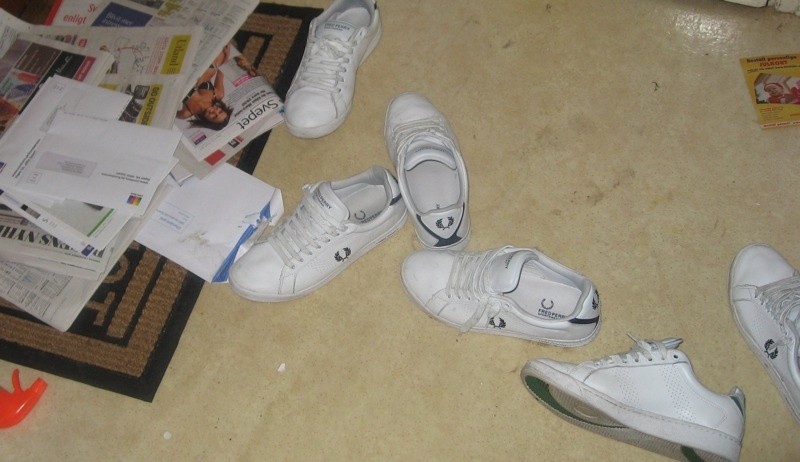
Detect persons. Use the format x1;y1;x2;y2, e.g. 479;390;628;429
232;54;258;78
177;44;232;131
763;83;798;104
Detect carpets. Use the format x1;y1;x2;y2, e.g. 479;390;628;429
0;0;323;403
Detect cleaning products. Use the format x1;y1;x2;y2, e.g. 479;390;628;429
0;367;48;429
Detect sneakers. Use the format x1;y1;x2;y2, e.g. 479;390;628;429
284;0;382;138
227;163;407;302
384;95;472;252
728;242;800;431
400;248;600;348
520;333;745;461
